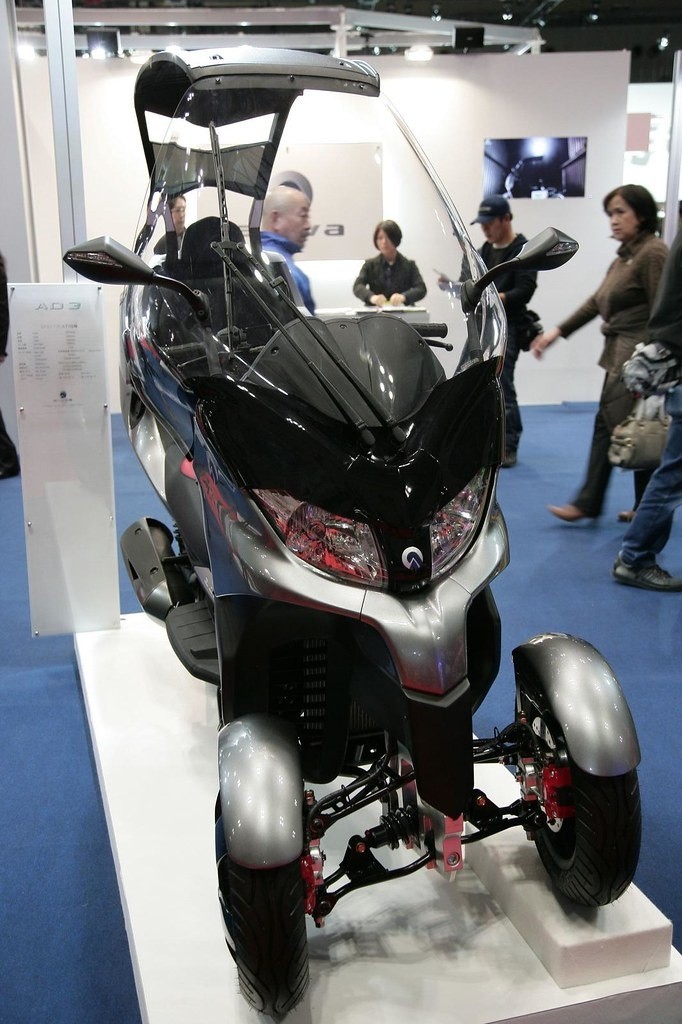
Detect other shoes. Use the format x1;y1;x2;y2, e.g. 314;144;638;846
619;509;638;521
548;503;599;521
503;448;519;467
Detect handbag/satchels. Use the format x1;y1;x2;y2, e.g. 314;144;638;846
515;309;542;351
608;393;672;468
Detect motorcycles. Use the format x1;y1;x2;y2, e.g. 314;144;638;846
58;45;643;1023
506;156;565;198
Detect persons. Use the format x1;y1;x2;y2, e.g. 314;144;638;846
0;254;21;478
439;194;540;468
612;223;682;593
535;184;670;524
353;220;427;307
153;196;186;256
258;186;315;318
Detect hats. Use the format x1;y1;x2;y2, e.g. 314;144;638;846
469;195;510;225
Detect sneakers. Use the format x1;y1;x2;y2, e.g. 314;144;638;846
613;551;682;591
